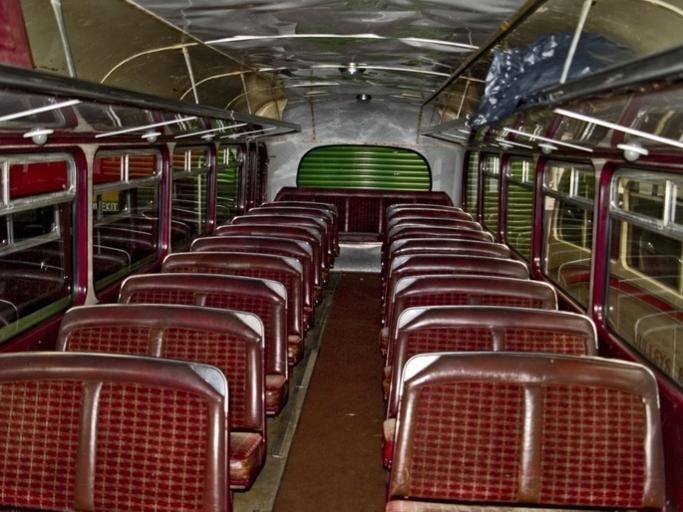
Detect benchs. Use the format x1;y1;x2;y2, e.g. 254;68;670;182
376;202;665;511
116;270;293;419
2;347;232;511
51;299;272;495
116;202;343;367
